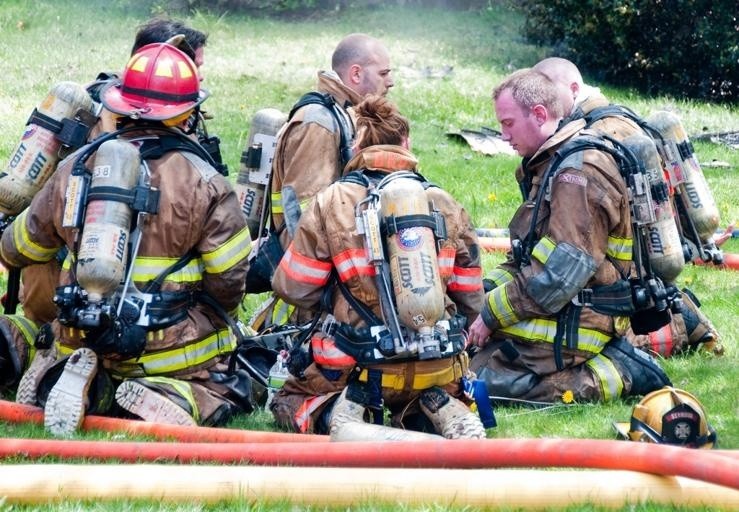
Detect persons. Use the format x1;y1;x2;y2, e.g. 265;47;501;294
3;18;735;441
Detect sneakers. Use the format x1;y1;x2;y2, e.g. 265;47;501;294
329;385;370;439
603;338;672;395
418;385;486;441
674;288;724;355
114;380;197;428
247;291;279;331
43;348;99;436
16;345;57;404
0;317;27;377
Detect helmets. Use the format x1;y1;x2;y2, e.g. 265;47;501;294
99;43;210;121
612;386;715;449
